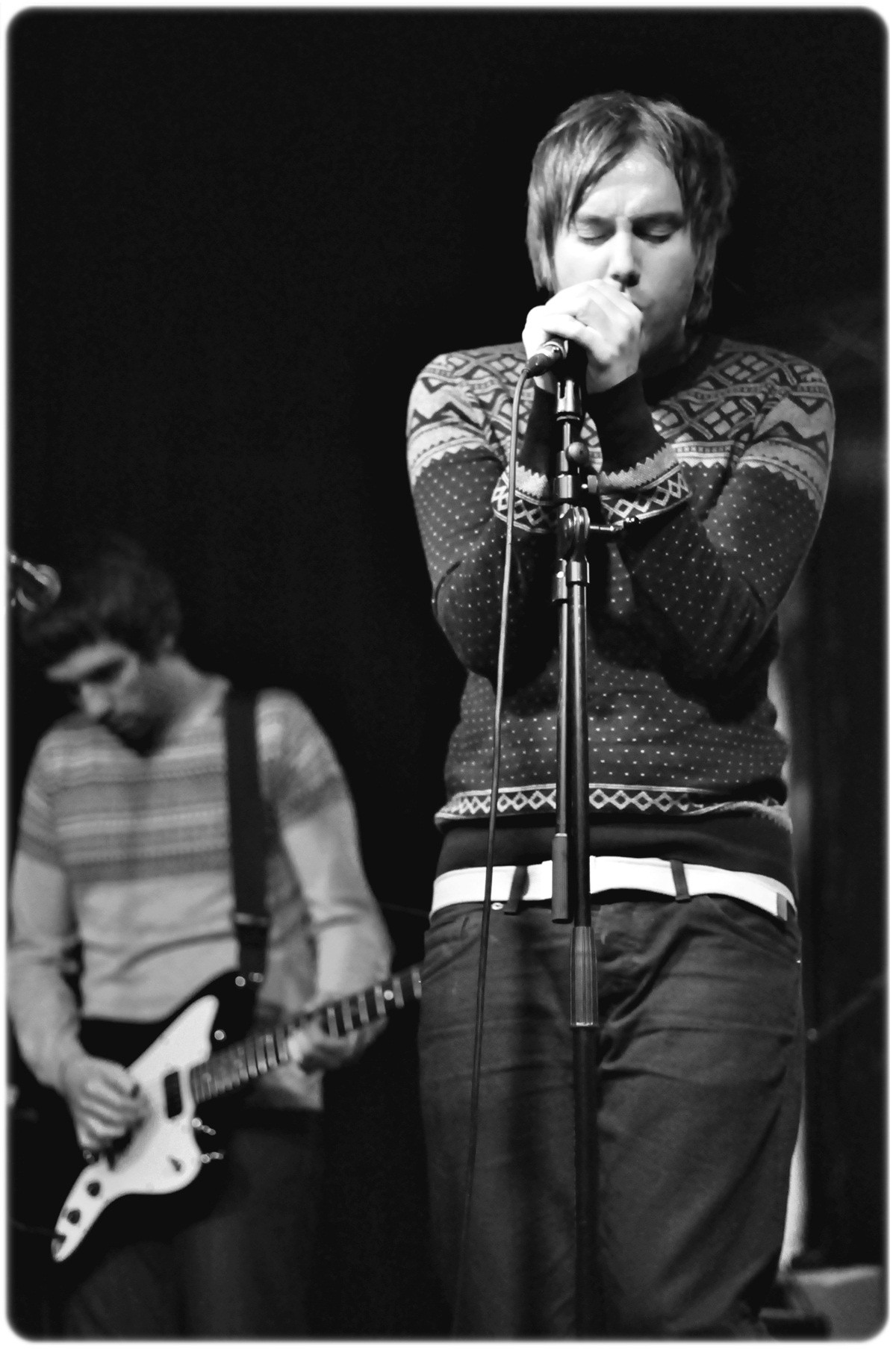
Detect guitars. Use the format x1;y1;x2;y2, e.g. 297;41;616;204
49;961;422;1265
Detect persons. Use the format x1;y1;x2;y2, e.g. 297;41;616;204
9;536;395;1340
405;96;837;1341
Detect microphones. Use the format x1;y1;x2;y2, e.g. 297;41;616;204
7;551;62;612
522;335;585;376
